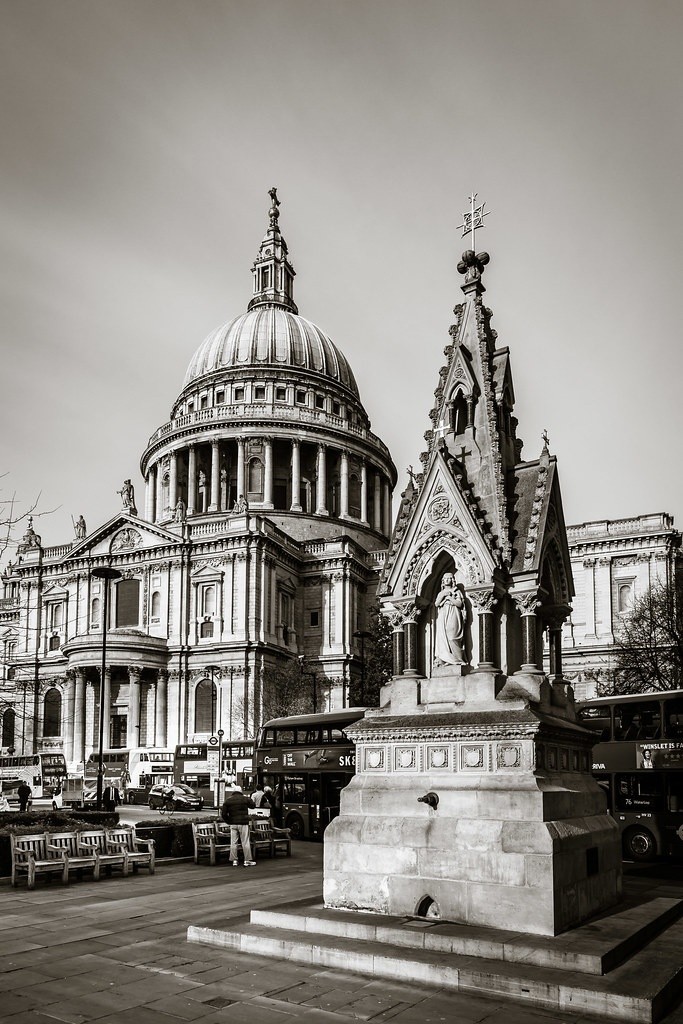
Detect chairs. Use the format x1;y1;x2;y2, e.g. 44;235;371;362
10;825;157;890
191;817;293;868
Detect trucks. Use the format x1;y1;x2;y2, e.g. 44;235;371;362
49;775;124;812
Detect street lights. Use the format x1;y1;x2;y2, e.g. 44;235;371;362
91;559;123;811
351;630;371;706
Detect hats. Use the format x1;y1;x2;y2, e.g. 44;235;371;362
23;781;27;783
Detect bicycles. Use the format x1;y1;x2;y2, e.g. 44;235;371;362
158;795;175;816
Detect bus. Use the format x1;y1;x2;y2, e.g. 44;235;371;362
173;737;305;813
0;752;70;811
241;689;683;865
81;746;175;806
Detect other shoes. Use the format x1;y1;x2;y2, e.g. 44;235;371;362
244;861;256;866
233;861;239;866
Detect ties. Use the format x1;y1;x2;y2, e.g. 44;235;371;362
111;787;113;799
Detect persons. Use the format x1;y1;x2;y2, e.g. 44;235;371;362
641;750;656;769
73;515;86;539
162;785;175;810
119;763;132;796
104;782;121;812
17;781;33;811
222;786;277;866
232;494;248;515
434;573;469;667
116;480;133;508
173;497;185;523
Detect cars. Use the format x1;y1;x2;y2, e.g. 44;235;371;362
148;782;205;812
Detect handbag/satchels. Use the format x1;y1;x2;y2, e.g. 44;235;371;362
263;796;272;808
